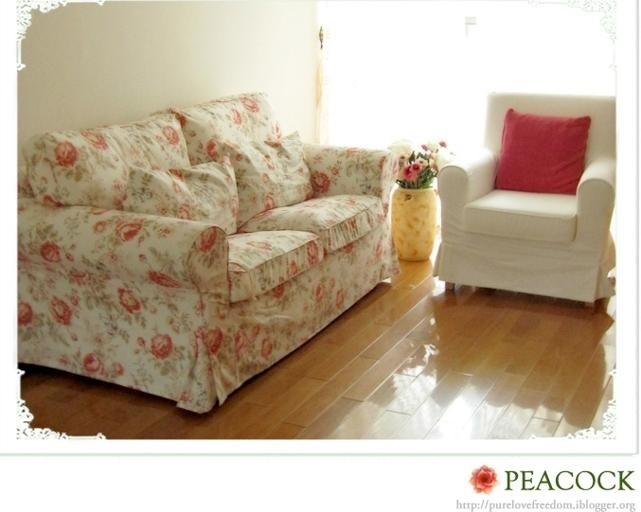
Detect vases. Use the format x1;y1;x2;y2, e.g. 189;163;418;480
391;186;437;262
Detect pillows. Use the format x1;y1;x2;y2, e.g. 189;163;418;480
203;120;314;230
494;108;591;195
124;152;241;237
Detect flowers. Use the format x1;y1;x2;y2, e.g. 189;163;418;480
393;140;447;189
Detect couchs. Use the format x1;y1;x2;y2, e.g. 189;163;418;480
17;90;398;414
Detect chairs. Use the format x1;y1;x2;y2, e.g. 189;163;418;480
432;90;616;310
